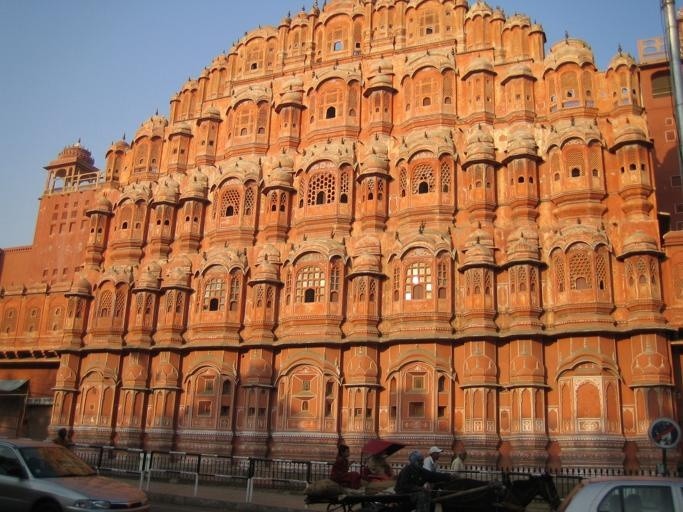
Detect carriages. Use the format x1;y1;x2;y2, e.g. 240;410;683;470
302;465;564;511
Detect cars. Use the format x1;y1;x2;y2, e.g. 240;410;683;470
555;474;683;512
0;435;147;511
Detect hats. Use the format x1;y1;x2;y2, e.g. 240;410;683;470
430;447;442;454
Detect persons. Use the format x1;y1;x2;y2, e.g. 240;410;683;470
421;446;443;472
394;451;454;512
366;452;389;479
52;427;68;449
450;452;468;481
330;444;360;491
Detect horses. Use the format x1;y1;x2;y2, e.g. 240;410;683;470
429;471;562;512
